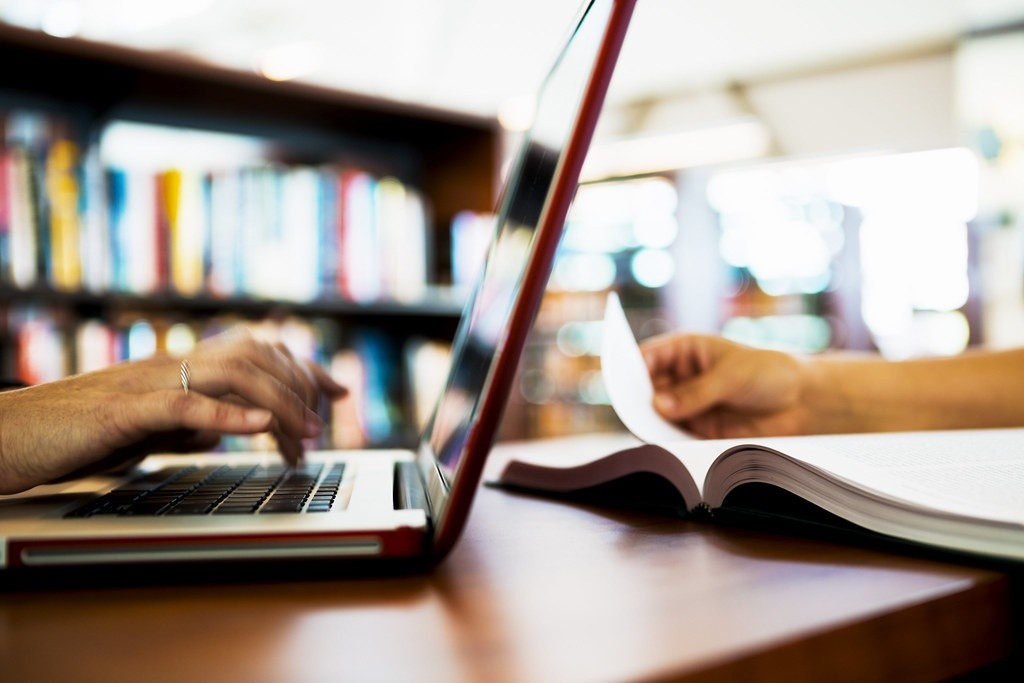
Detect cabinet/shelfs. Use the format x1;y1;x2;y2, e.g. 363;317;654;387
0;23;505;451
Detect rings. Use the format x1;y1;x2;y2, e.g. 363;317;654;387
180;360;189;392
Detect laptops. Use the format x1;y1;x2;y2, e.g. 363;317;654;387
0;0;640;580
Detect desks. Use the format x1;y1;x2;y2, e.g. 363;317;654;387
0;432;1008;683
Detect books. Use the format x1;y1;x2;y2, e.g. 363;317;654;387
478;289;1024;567
0;116;428;448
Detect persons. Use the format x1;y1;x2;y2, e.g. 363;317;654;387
0;333;347;501
636;333;1024;441
